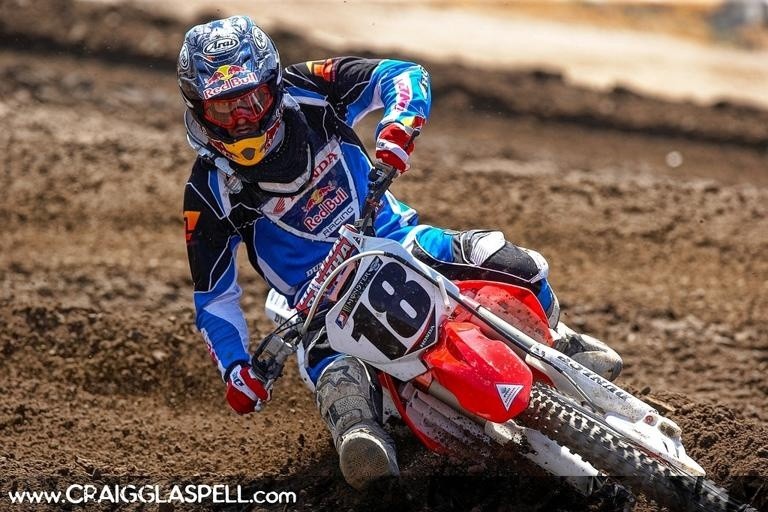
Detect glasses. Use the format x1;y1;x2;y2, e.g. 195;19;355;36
203;83;274;129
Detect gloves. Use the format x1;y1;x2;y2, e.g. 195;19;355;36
375;122;414;175
224;365;268;416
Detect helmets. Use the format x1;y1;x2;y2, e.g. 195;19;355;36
176;15;286;166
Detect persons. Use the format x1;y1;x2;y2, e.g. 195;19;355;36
177;16;623;491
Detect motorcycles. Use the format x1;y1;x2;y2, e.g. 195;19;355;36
249;128;760;512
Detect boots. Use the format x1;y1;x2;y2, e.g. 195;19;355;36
315;354;401;498
549;320;623;382
